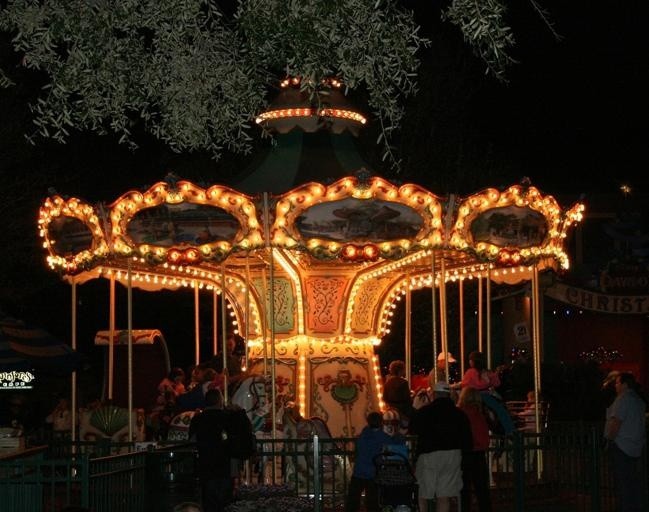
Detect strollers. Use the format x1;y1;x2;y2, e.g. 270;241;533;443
373;450;418;512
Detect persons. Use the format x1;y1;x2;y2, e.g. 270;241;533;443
383;362;417;416
431;352;534;402
44;333;257;512
409;383;473;510
605;373;646;509
461;389;493;510
348;412;413;510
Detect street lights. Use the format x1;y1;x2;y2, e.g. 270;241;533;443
619;181;633;250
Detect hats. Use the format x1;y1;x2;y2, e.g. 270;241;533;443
433;382;450;392
438;352;456;363
